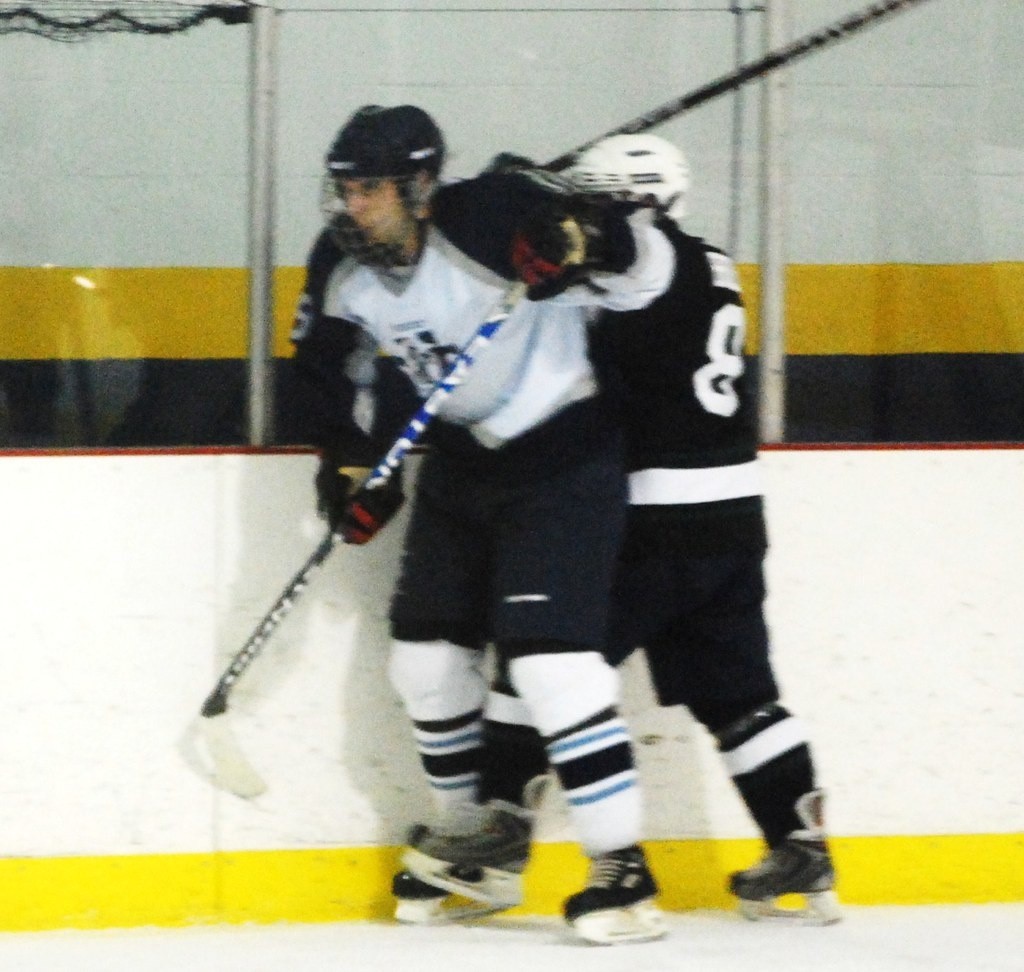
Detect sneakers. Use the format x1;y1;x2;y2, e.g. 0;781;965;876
393;873;508;922
723;791;840;924
399;776;549;905
565;844;669;943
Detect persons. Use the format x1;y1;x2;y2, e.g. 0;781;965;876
291;104;676;945
405;130;842;927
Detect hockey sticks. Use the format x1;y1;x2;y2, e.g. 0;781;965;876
187;280;527;802
550;1;918;163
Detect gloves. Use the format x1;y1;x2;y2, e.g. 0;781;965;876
316;446;407;543
511;214;635;302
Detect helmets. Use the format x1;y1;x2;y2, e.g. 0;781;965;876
573;133;693;217
320;103;443;267
